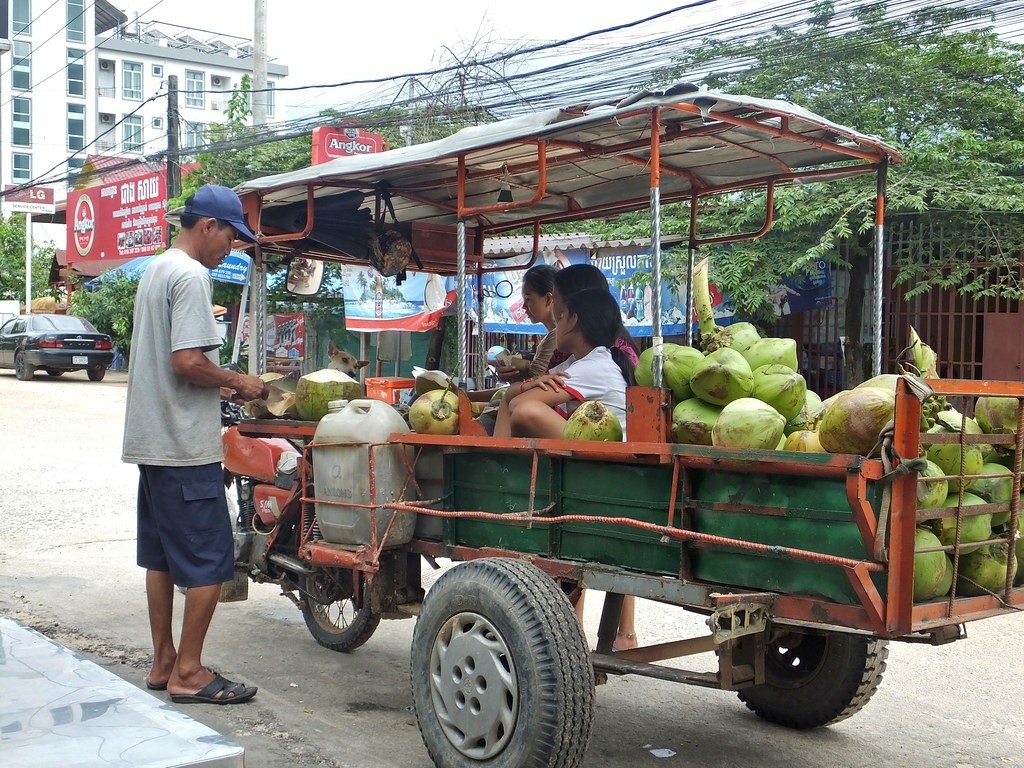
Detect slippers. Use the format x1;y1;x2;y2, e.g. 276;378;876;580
170;671;257;703
145;666;221;690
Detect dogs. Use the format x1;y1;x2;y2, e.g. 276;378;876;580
326;339;370;379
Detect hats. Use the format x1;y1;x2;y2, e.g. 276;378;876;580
185;183;259;244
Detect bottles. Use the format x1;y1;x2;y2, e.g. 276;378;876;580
644;280;652;318
374;275;383;318
627;283;635;318
620;284;627;315
635;282;644;321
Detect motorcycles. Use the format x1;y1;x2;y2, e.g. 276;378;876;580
221;87;1024;768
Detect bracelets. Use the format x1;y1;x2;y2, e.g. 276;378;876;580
520;380;531;394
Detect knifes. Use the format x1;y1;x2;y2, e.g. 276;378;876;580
231;370;300;401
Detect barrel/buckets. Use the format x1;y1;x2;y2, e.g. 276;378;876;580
312;399;417;545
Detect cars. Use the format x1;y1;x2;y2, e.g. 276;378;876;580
1;313;116;382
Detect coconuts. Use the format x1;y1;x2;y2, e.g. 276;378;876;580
561;398;623;442
489;388;508;402
408;371;460;434
294;368;362;420
633;322;1024;603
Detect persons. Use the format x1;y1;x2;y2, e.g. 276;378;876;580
121;184;271;704
505;288;626;442
545;264;639;651
486;264;557;384
